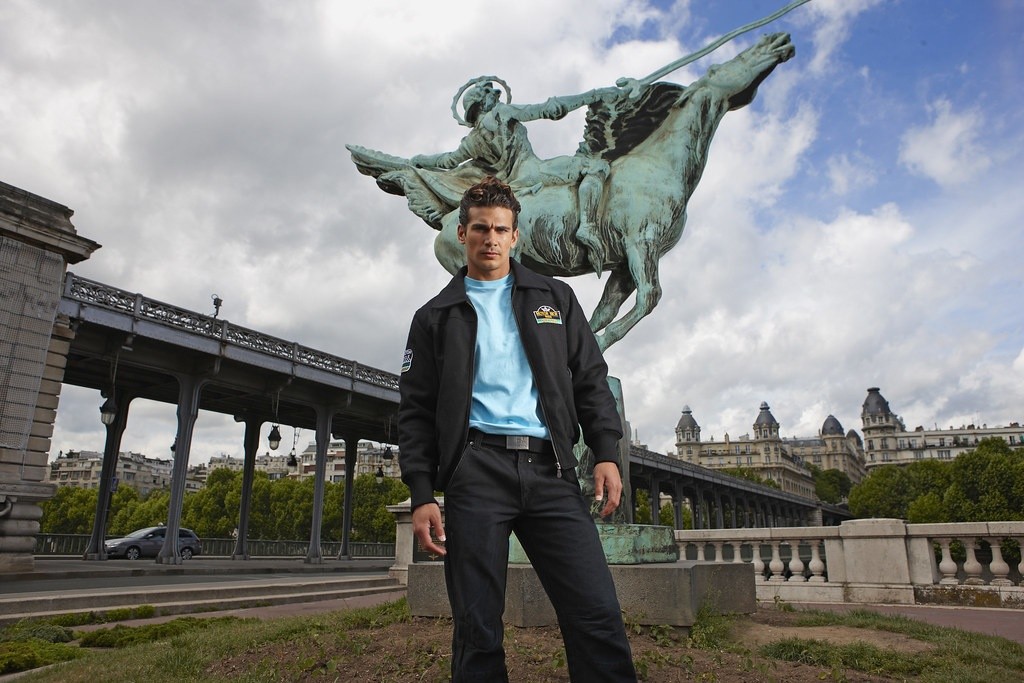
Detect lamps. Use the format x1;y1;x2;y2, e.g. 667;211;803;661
375;442;384;484
100;348;119;424
170;445;175;457
268;391;282;450
383;413;394;467
288;426;300;473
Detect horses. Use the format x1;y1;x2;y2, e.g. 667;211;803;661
350;32;797;355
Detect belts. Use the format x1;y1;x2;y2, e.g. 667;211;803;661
467;430;554;454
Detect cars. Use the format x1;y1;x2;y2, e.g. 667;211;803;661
103;522;204;561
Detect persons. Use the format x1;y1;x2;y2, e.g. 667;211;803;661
399;179;640;683
411;76;620;249
